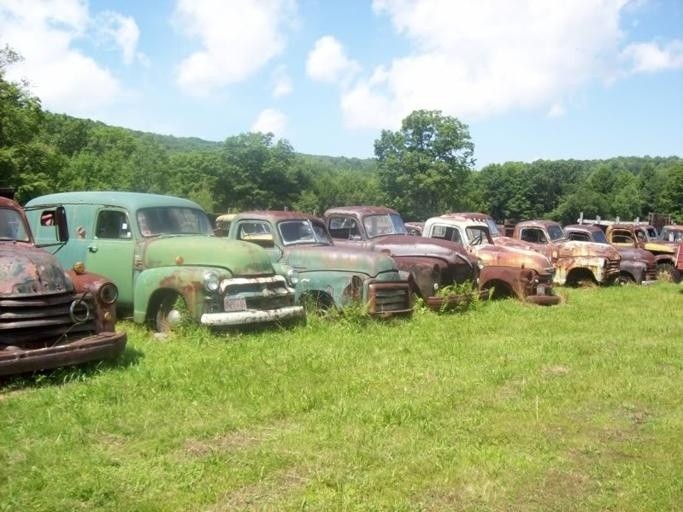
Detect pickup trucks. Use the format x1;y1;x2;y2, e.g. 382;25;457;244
420;211;561;308
320;205;489;309
495;220;621;288
602;220;680;285
214;210;413;321
561;224;646;287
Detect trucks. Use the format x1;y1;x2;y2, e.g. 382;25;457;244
575;211;682;270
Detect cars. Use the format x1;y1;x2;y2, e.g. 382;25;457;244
17;191;305;335
0;195;126;375
